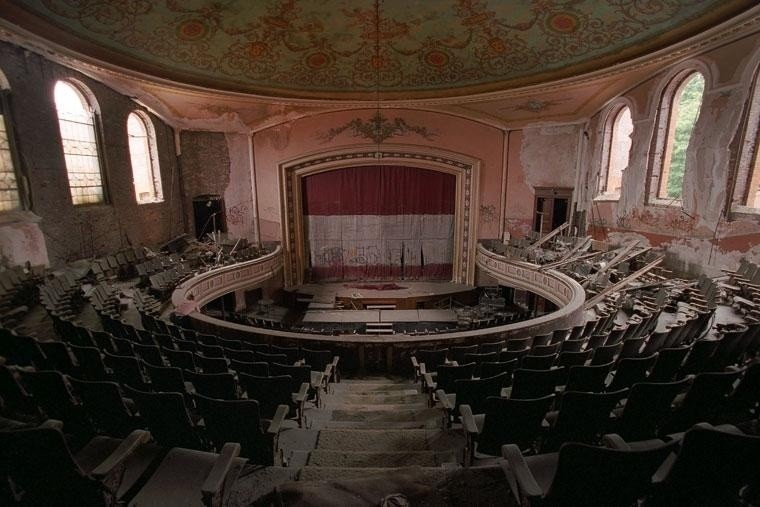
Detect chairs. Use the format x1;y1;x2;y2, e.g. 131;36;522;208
0;247;340;503
404;259;759;505
